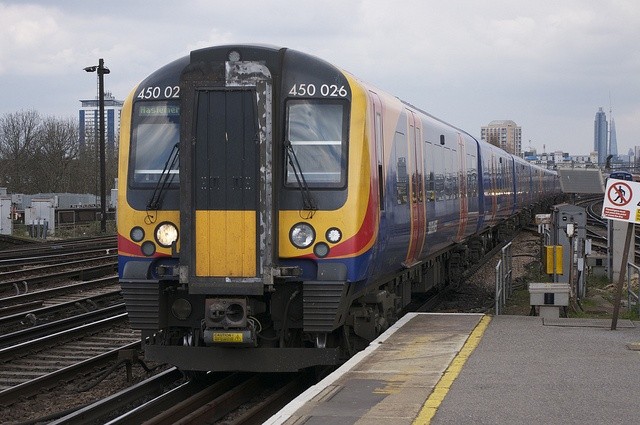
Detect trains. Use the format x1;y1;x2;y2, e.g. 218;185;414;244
118;44;561;381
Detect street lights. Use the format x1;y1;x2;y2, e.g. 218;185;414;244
84;57;111;233
